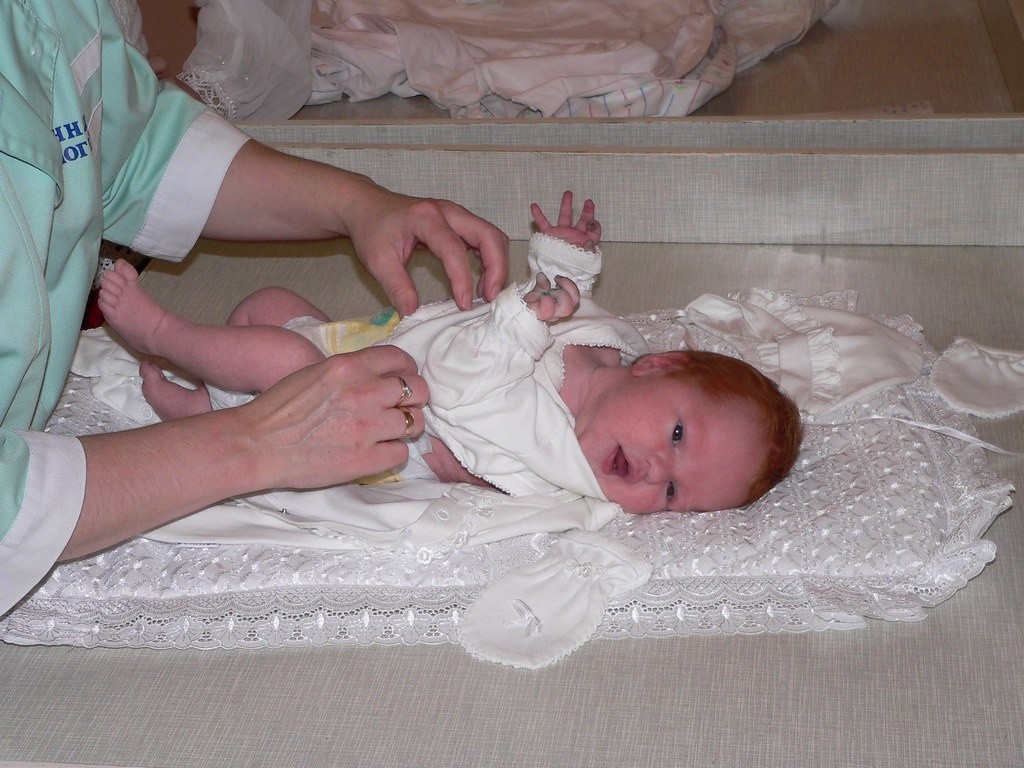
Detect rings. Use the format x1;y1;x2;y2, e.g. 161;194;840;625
382;373;413;408
395;407;415;440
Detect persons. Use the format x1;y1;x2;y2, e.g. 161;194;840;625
99;190;806;516
1;0;509;618
